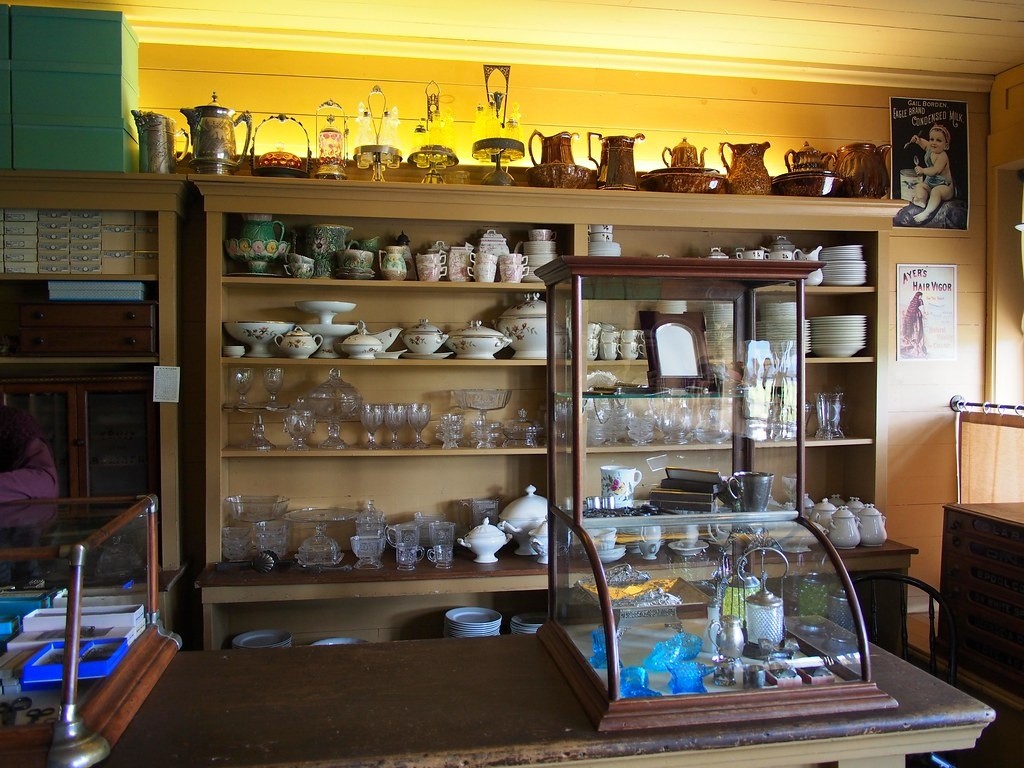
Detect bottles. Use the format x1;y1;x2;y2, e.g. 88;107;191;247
379;229;415;280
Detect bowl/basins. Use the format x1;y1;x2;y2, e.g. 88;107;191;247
642;173;732;201
222;495;290;561
525;165;593;189
775;171;847;196
274;292;546;358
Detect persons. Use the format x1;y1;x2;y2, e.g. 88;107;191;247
0;406;59;586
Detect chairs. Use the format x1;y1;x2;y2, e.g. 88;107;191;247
829;571;956;685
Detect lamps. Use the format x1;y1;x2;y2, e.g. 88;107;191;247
314;64;525;186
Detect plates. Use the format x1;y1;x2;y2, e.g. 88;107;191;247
657;303;866;357
223;345;245;357
232;605;544;647
401;352;453;359
819;245;867;287
590;542;709;564
525;241;621;282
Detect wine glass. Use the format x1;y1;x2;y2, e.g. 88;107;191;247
351;535;453;571
236;366;432;450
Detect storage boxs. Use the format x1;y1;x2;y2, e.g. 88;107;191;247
0;209;158;275
0;5;140;172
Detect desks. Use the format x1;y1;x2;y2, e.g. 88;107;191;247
98;613;999;768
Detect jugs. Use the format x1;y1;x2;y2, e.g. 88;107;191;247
348;235;379;278
527;128;835;190
761;236;823;286
131;93;253;173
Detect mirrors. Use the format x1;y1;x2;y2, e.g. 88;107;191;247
637;309;722;392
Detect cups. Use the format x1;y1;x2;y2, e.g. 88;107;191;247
587;225;615;241
600;464;772;511
800;491;886;547
284;249;374;278
417;252;527;282
735;248;793;262
638;526;661;560
385;521;455;548
587;321;647;359
529;228;557;239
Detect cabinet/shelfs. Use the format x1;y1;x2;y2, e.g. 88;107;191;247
191;173;918;731
936;503;1024;679
0;172;190;637
